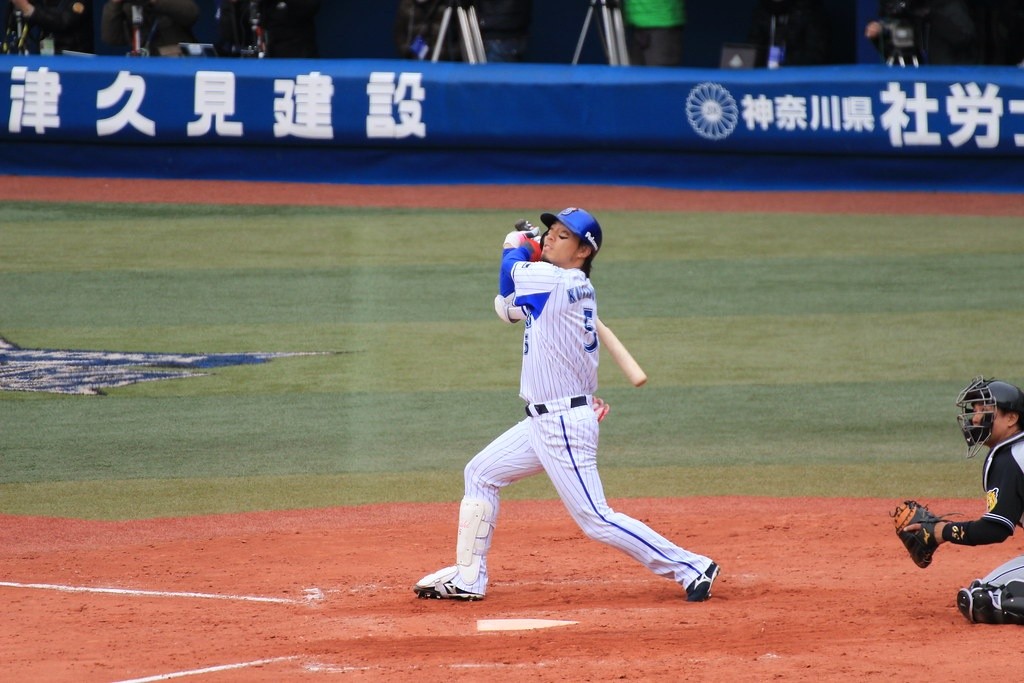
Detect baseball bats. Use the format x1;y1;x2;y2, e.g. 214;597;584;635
514;219;647;388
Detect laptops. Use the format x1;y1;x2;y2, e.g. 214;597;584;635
177;42;218;58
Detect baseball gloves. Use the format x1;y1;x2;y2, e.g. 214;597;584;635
889;499;949;570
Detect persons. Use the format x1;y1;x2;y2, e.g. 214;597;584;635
100;0;201;56
218;1;321;57
5;0;94;53
394;0;532;63
865;0;983;66
412;206;720;604
894;380;1024;624
746;0;856;69
623;0;688;65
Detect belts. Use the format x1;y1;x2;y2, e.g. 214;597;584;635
525;395;587;416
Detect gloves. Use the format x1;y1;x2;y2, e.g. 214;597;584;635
592;395;611;424
527;235;541;261
503;221;539;249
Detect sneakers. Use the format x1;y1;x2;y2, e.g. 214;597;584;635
414;582;483;600
687;561;721;601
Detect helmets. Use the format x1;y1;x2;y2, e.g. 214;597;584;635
540;207;602;252
971;380;1024;416
